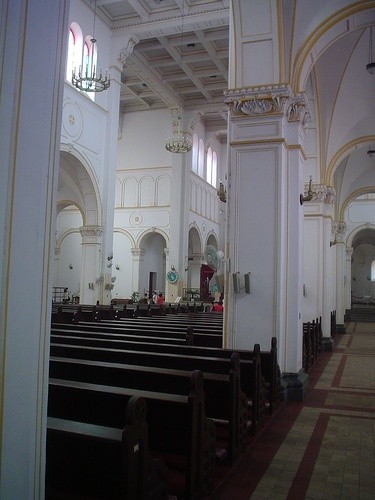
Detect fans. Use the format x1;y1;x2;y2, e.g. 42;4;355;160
203;244;230;274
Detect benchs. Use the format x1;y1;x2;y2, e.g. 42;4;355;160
44;300;338;500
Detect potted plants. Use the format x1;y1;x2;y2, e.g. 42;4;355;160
186;290;199;301
131;292;139;304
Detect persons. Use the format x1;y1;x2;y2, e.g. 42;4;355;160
63;287;71;304
214;300;223;311
142;290;164;305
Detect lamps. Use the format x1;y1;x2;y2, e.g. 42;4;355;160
367;150;375;158
165;1;194;153
217;182;228;202
364;24;375;75
300;175;313;205
330;233;338;247
72;1;112;92
